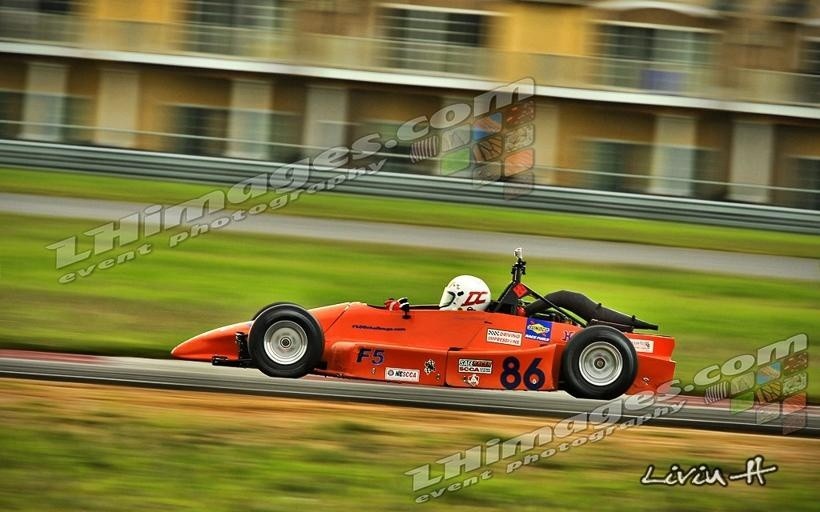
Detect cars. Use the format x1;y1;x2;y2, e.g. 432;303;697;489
171;247;677;400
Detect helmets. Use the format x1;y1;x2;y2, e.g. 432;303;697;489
439;275;492;310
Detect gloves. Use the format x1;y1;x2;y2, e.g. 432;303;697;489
384;300;401;311
